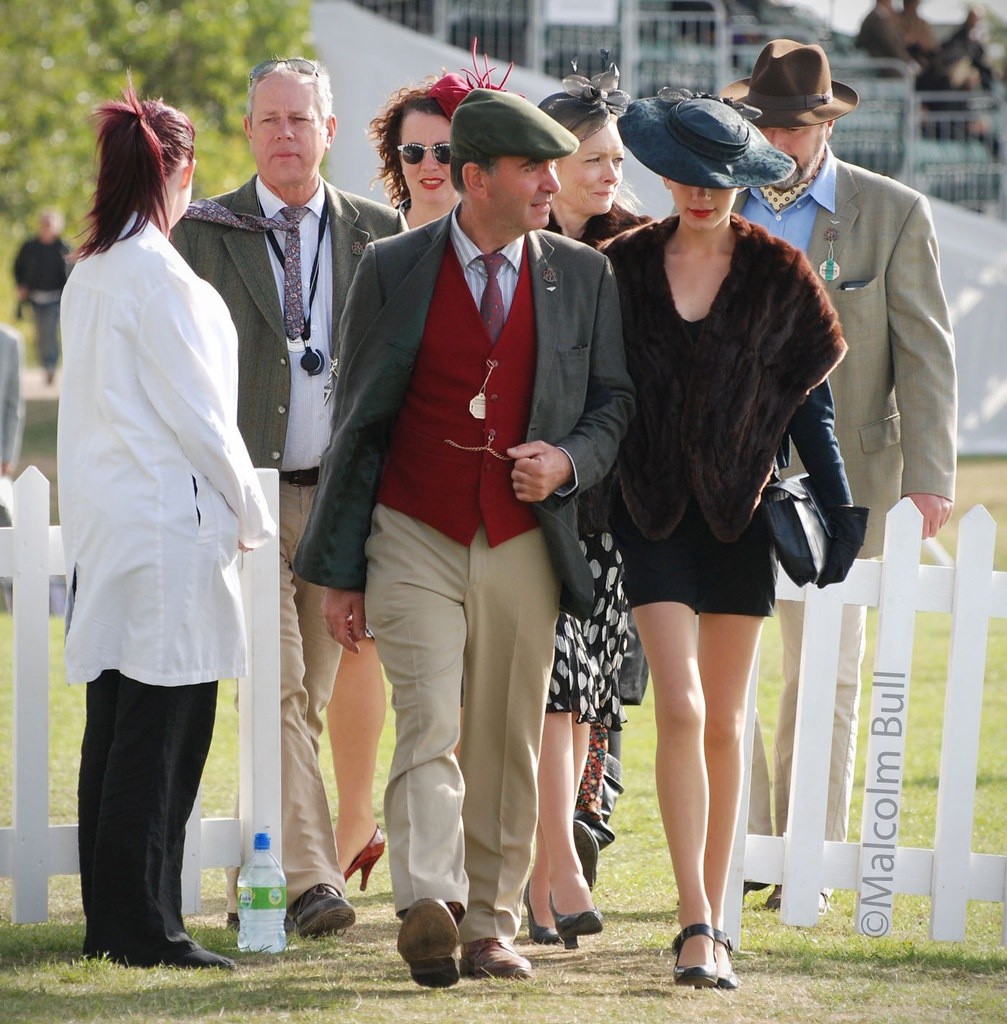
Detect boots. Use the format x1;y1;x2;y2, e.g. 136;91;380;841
573;752;625;891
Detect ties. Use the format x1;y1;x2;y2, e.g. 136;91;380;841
183;198;311;339
480;251;509;346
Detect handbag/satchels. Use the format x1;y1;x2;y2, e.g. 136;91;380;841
761;473;831;586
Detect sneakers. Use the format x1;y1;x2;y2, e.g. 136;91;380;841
287;884;357;938
228;912;294;934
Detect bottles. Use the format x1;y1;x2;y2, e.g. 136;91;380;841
237;833;287;954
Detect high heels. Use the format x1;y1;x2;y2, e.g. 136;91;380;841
671;924;719;991
343;824;386;891
713;928;739;990
550;893;604;950
524;879;563;946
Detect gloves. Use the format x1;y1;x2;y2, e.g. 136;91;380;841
817;508;869;588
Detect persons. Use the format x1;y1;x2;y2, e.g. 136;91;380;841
577;84;869;987
330;37;655;952
163;57;422;938
292;84;637;988
672;0;1007;162
58;67;279;972
0;208;74;609
719;37;957;908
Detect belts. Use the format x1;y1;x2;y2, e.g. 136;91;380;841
280;467;319;487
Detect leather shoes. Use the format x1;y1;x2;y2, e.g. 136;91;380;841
458;938;534;980
397;898;460;988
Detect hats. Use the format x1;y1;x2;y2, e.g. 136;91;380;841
719;39;859;127
618;86;797;188
451;88;579;159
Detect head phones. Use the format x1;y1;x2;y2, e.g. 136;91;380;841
300;346;325;376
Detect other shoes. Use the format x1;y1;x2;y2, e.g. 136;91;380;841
174;948;239;970
764;885;782;909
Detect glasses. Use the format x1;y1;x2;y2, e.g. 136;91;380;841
397;144;452;165
